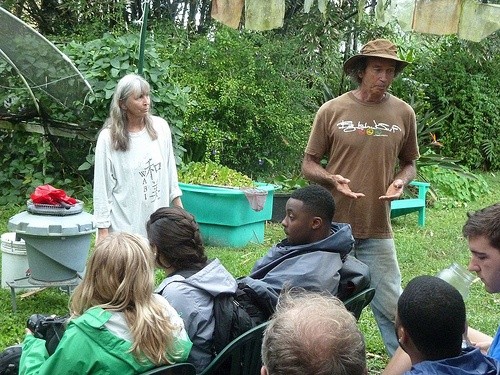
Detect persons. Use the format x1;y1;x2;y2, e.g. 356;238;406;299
301;38;419;356
146;205;238;375
93;73;184;245
261;281;367;375
19;232;193;374
236;183;370;317
380;201;500;374
396;275;500;374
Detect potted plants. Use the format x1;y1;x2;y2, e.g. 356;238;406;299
257;175;308;223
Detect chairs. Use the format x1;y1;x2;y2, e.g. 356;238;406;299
344;287;376;321
199;319;274;375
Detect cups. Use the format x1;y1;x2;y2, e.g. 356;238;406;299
437;262;477;301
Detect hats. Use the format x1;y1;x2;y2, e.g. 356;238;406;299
343;39;412;74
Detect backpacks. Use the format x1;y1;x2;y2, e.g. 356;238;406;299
337;256;371;301
0;347;22;375
212;284;274;375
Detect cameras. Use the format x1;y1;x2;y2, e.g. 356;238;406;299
27;314;65;340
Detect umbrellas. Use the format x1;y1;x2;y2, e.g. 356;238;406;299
30;183;78;210
